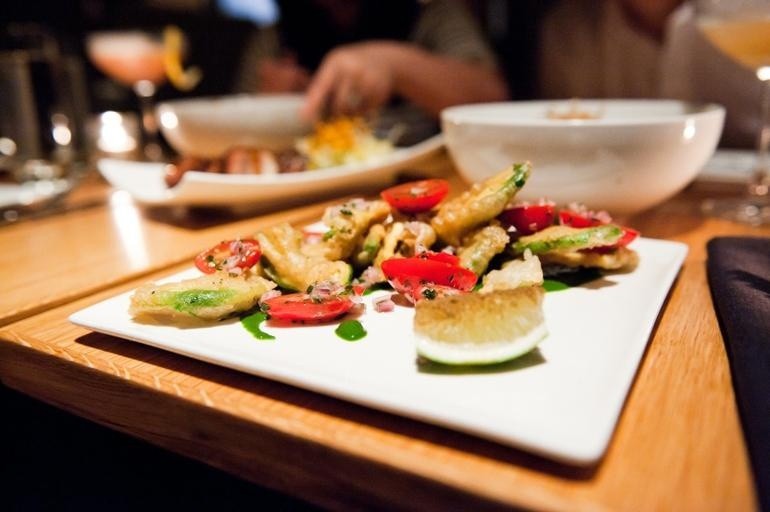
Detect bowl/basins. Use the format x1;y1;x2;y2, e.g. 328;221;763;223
435;98;727;224
158;93;320;160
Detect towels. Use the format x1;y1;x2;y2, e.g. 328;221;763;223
700;223;770;512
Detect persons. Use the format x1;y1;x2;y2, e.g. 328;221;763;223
152;2;507;158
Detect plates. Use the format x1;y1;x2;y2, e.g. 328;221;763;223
91;126;453;214
67;226;691;471
